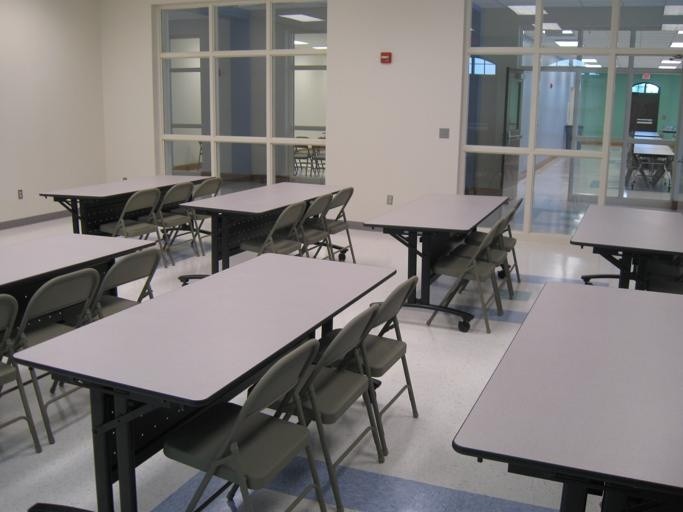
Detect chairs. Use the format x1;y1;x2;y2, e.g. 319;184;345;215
0;247;161;453
100;176;222;269
240;187;356;263
636;254;683;294
426;198;523;333
294;133;326;176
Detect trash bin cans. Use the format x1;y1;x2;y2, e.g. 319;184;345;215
565;125;583;150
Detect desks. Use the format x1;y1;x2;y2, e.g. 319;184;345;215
178;182;341;286
0;231;155;297
453;281;683;512
631;143;674;191
12;253;398;512
363;193;508;332
570;204;683;288
633;131;661;138
39;176;216;233
